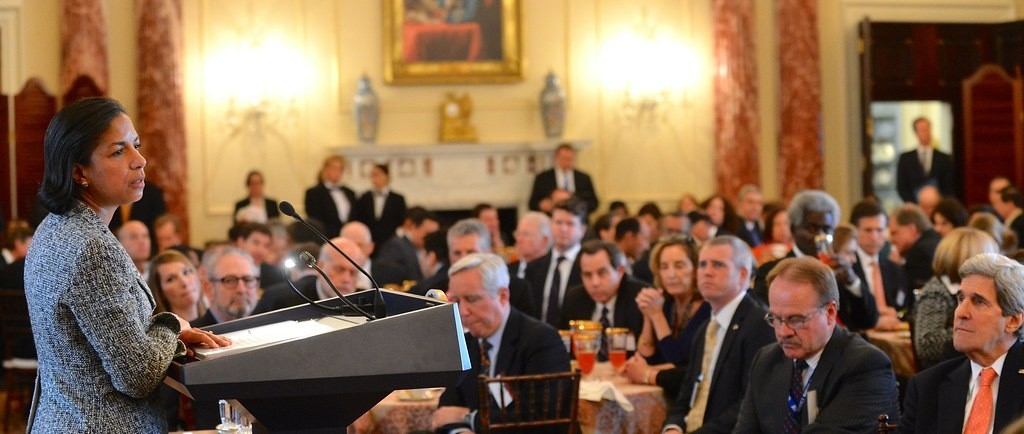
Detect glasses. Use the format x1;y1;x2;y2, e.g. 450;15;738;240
211;274;261;289
763;300;829;331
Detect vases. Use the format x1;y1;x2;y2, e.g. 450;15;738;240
353;75;380;145
540;72;567;141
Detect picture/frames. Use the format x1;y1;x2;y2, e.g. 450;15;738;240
379;0;529;89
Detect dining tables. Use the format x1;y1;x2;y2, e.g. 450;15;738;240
345;358;671;434
859;321;919;378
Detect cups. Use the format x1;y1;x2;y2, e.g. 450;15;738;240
606;328;628;370
815;233;838;268
558;320;602;375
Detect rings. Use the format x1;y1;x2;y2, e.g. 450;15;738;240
209;330;214;334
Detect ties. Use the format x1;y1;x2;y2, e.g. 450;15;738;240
871;261;887;310
685;317;722;431
478;338;494;424
783;359;809;434
963;367;997;434
597;307;611;362
545;256;567;327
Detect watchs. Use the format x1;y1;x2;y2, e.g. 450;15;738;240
462;408;474;424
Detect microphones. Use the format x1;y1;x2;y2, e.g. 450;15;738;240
298;251;376;323
279;201;386;319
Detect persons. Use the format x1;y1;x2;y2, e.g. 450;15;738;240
25;96;232;434
0;144;1024;434
897;118;956;204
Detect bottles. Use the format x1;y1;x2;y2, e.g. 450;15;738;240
354;78;378;144
215;400;252;434
540;75;565;138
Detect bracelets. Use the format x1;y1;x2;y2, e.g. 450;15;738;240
642;369;651;386
